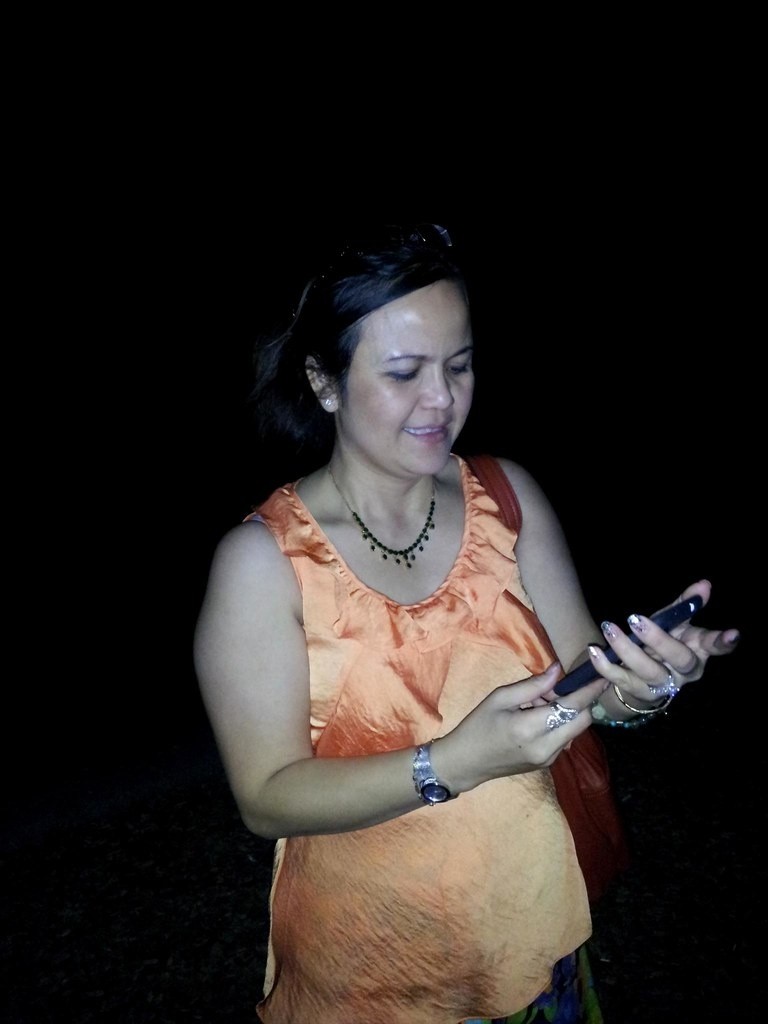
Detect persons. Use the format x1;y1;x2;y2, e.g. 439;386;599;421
193;216;740;1024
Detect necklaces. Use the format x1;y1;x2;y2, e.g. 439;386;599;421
330;467;438;567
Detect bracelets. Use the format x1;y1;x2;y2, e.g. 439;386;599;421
589;680;675;729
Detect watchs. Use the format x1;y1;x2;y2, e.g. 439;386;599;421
412;739;459;807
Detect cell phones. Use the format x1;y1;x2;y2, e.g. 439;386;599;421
553;595;704;697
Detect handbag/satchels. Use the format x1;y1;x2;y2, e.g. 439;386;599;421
466;452;627;898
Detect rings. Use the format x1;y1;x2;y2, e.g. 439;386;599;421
545;700;579;733
648;666;679;697
564;739;573;750
